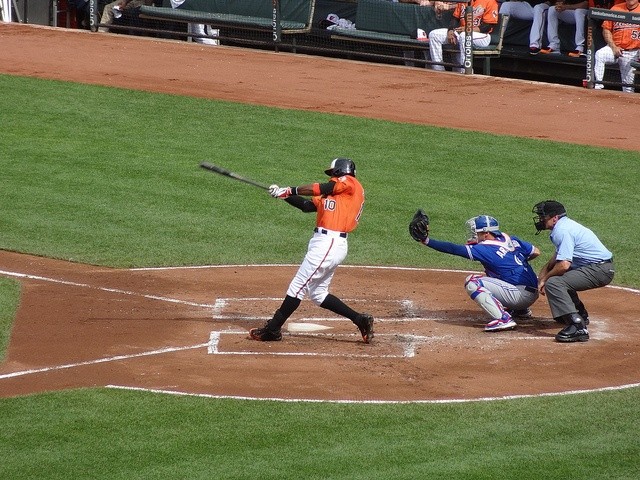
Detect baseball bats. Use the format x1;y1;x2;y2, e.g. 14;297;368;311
201;162;271;190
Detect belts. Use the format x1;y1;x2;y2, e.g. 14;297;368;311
525;286;535;293
314;227;346;238
602;258;613;263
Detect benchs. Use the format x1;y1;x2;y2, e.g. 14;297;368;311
137;1;316;53
329;0;510;75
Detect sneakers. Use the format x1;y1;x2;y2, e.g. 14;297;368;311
483;319;518;332
249;319;282;341
519;311;532;317
353;314;374;344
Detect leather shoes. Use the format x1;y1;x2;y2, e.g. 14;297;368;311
556;325;589;342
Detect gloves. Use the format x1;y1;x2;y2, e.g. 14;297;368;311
269;185;298;199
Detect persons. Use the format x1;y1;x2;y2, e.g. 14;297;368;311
531;199;617;344
540;0;595;58
589;0;639;93
495;0;549;55
96;1;147;33
407;208;541;332
426;0;499;74
248;156;377;345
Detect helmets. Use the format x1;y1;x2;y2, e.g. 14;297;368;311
532;201;566;235
464;215;499;242
324;158;356;177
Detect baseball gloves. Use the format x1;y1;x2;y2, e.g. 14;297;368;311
409;208;429;242
